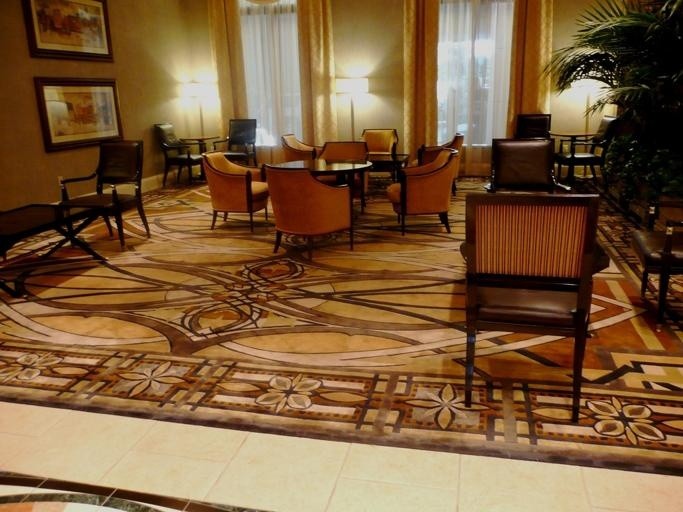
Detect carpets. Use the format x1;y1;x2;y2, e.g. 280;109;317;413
0;166;682;480
0;468;273;512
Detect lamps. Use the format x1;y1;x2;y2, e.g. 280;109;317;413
333;75;371;141
565;78;605;176
175;81;217;138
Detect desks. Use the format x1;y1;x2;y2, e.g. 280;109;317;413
0;201;106;298
461;238;610;282
179;134;221;184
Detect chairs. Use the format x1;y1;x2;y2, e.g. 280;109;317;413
511;111;553;140
211;117;257;170
153;122;206;191
260;162;356;261
200;150;269;234
629;197;683;324
556;117;616;177
278;122;467;238
461;187;606;422
55;137;151;255
490;140;558;191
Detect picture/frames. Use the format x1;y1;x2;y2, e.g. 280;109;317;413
19;0;115;65
30;72;124;155
